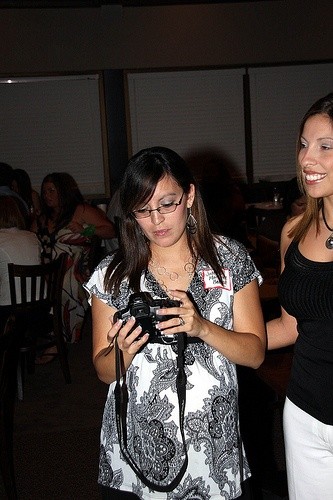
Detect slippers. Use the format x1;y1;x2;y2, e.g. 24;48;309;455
33;351;58;365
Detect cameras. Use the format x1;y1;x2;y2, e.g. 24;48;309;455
116;291;179;345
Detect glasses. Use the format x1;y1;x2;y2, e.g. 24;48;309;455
130;190;185;220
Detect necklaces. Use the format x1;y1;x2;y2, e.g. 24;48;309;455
150;253;194;294
321;205;333;250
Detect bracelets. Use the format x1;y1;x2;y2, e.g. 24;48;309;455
80;222;96;236
271;240;274;248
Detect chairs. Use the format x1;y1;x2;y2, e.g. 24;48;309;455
236;177;303;217
246;207;281;233
256;234;294;361
0;253;71;499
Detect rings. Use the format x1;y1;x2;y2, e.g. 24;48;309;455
179;317;184;326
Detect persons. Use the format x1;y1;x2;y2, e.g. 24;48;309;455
0;160;307;365
263;92;333;500
86;145;267;500
